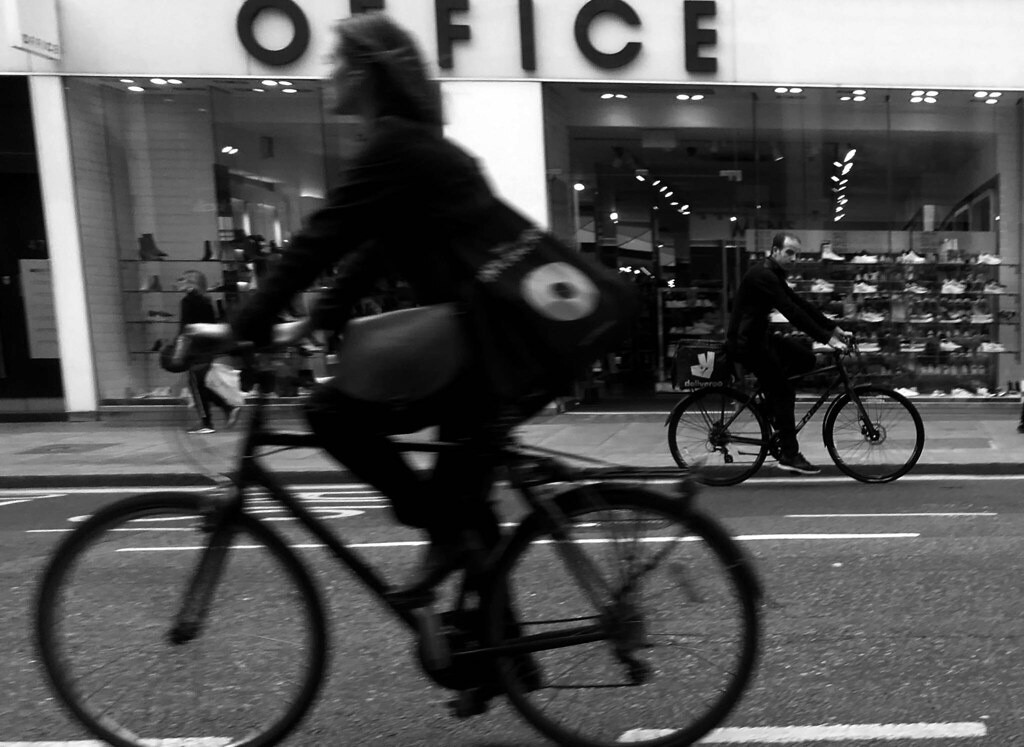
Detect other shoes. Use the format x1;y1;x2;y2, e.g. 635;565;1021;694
382;507;499;609
225;407;239;429
451;647;542;718
658;289;721;388
186;422;216;434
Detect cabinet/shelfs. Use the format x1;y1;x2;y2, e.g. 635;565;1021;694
120;257;264;355
656;240;750;391
765;257;1022;401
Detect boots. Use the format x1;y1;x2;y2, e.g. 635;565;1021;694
125;233;333;400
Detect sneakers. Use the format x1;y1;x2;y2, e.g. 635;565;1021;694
777;453;821;474
758;242;1016;399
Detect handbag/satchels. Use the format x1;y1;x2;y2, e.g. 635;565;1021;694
159;332;189;373
460;226;639;380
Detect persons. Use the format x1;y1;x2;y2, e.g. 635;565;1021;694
732;233;853;474
177;270;241;434
181;10;593;718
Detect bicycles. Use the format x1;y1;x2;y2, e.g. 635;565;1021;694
31;271;766;747
663;330;926;490
